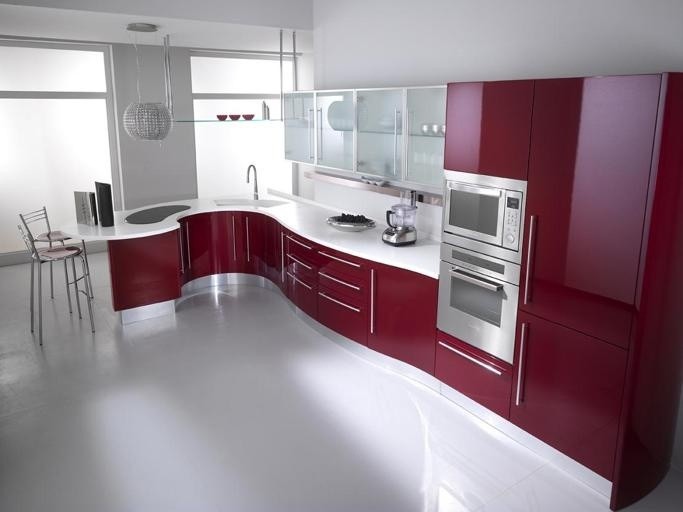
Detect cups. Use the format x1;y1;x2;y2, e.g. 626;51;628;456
420;122;445;135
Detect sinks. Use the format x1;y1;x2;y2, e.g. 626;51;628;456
213;199;289;208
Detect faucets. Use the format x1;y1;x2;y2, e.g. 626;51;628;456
247;165;258;201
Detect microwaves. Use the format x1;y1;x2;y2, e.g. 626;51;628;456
441;169;528;266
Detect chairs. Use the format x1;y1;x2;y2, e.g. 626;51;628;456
18;226;95;345
20;205;96;313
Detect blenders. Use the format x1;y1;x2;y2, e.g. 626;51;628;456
382;204;418;247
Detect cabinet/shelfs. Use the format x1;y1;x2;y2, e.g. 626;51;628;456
284;85;447;196
437;72;683;512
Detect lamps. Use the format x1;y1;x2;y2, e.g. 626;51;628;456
123;23;172;143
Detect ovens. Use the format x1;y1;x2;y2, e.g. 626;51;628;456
436;242;521;366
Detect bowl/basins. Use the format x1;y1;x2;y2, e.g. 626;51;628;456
242;115;254;120
217;115;228;121
378;115;402;132
229;115;240;120
